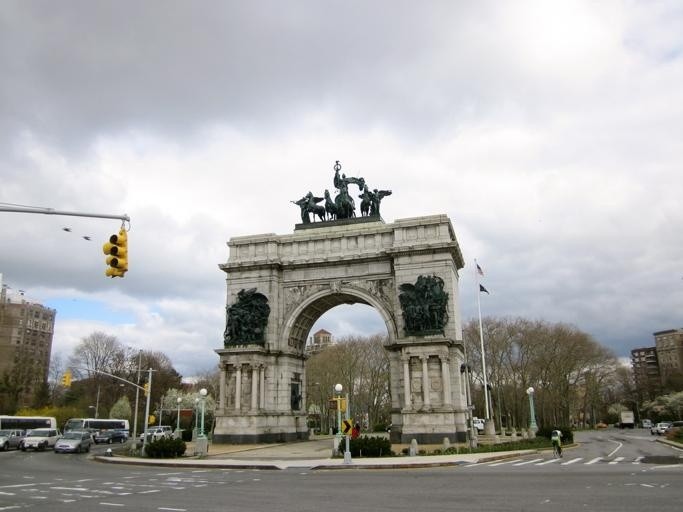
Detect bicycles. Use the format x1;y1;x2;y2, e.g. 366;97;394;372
551;442;564;457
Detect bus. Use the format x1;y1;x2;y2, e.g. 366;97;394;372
0;415;56;429
64;418;129;433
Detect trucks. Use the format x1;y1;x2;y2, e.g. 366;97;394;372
617;410;635;429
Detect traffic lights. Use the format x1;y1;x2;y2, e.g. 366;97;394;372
61;369;73;388
102;228;128;279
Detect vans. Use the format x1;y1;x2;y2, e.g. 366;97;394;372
473;416;485;432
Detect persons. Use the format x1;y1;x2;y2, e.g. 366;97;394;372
351;424;359;438
550;428;564;458
289;161;391;224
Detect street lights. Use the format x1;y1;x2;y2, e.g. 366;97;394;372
333;381;346;438
524;385;541;428
88;405;99;416
176;389;209;437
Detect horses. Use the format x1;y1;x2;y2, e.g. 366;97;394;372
301;191;326;221
359;184;375;216
325;188;337;220
337;182;353;218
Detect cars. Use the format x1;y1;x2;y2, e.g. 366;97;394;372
0;429;130;454
139;425;172;441
637;418;683;436
595;422;619;428
